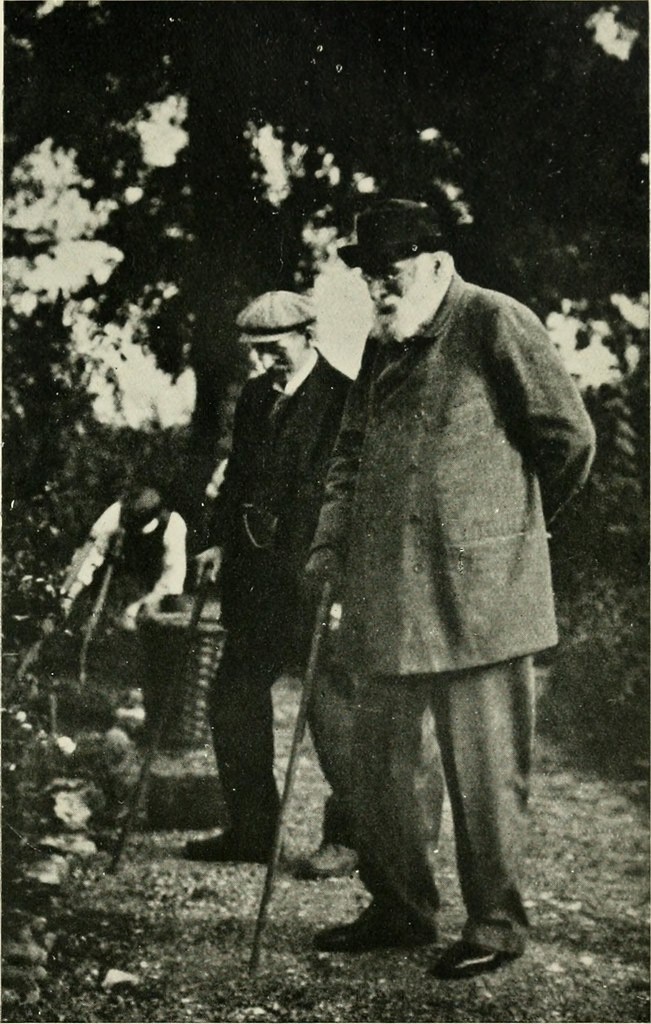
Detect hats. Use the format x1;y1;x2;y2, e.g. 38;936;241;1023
336;198;471;268
235;290;317;343
119;487;162;530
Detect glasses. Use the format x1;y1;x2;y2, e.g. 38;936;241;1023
362;259;429;283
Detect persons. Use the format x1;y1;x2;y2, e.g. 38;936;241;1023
304;198;595;980
181;291;357;878
38;486;187;635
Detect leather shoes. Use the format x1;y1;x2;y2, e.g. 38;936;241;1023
301;840;359;878
314;900;437;953
187;833;278;863
440;941;521;977
